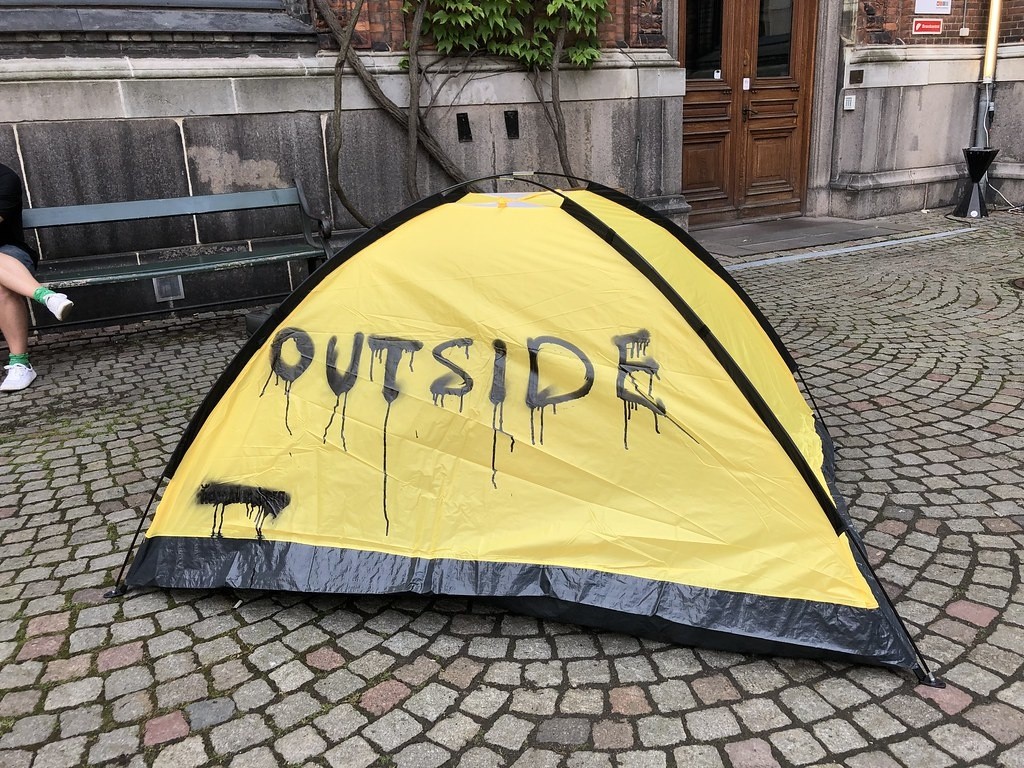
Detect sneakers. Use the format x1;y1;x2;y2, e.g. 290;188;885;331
44;294;74;322
0;362;37;391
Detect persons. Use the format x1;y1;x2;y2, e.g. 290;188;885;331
0;163;74;392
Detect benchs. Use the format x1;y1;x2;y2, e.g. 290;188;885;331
21;178;336;298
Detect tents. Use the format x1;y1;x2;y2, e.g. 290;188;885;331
110;173;948;691
